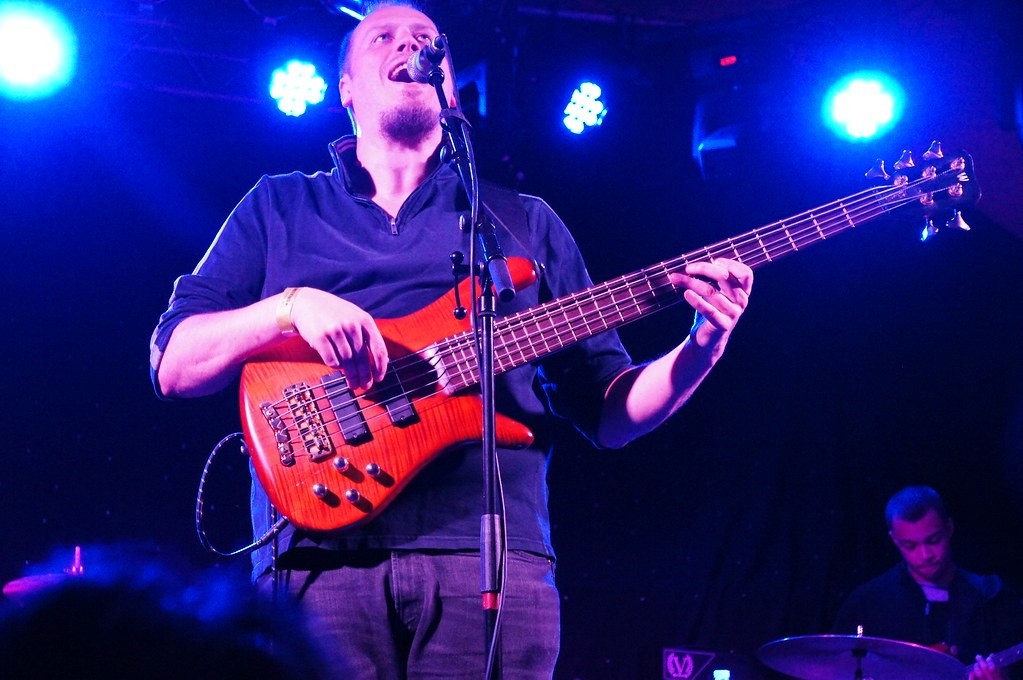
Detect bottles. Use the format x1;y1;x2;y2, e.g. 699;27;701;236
713;669;731;680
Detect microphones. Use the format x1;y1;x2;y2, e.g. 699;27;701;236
407;34;448;84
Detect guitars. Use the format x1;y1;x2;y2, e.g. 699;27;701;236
926;641;1023;680
239;139;984;540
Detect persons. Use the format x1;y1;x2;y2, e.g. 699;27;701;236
836;483;1023;679
147;3;752;680
0;540;361;680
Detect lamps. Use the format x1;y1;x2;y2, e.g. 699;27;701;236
688;47;776;187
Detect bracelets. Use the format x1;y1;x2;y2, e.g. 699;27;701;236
275;286;300;337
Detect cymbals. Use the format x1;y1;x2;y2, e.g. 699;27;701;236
755;634;964;680
